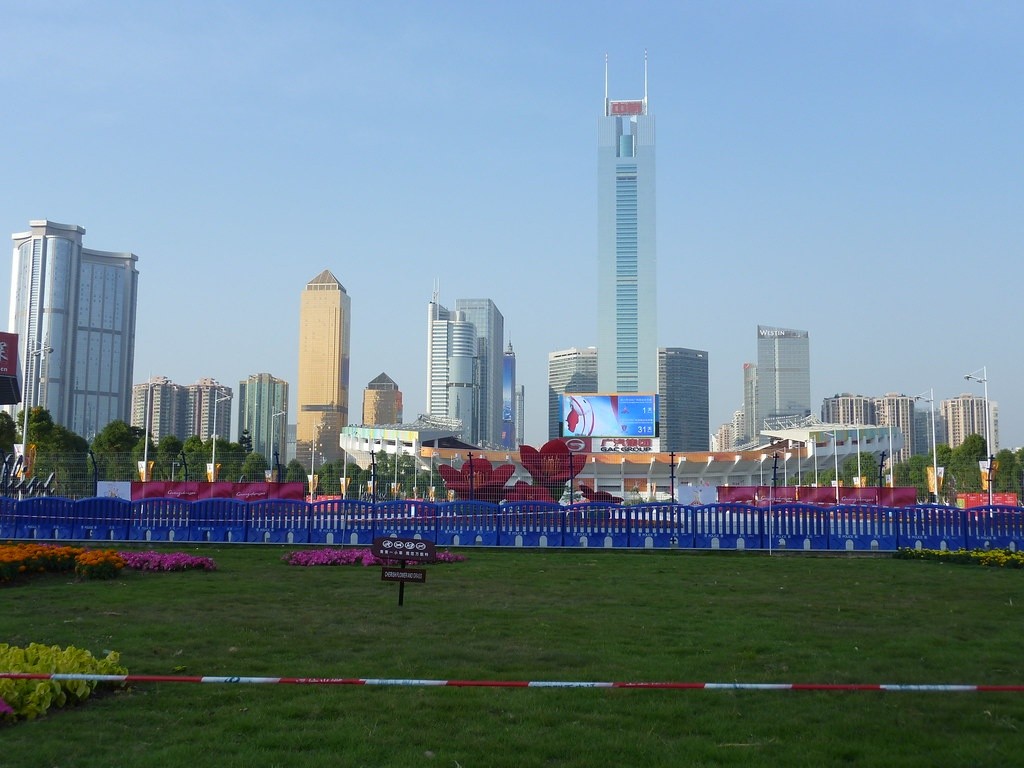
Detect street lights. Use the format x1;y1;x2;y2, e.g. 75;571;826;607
805;435;818;488
270;407;287;482
311;419;324;504
876;404;895;487
18;347;54;500
842;419;860;487
965;366;994;517
823;430;838;503
144;379;171;483
790;441;801;486
212;389;231;483
913;388;937;505
776;447;786;486
